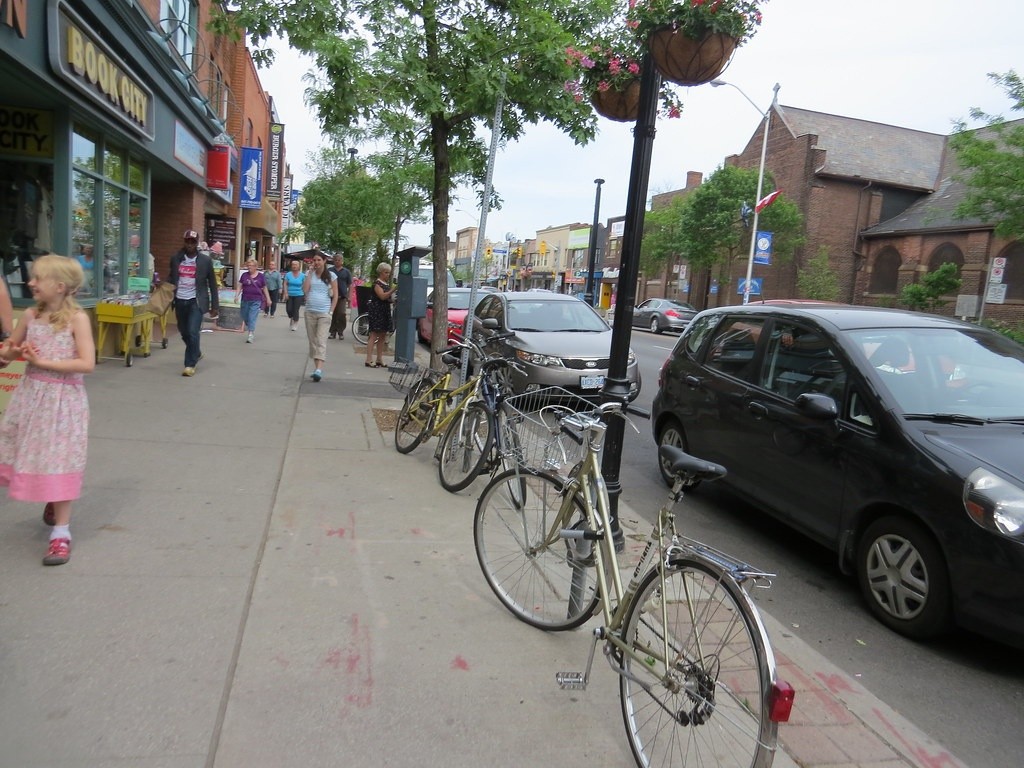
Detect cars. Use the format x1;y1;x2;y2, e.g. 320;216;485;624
633;298;700;334
415;286;642;413
651;303;1023;647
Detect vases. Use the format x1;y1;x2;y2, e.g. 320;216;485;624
647;23;737;87
590;74;642;123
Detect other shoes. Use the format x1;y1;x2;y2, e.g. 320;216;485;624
339;335;344;340
247;336;254;342
329;334;336;339
311;370;322;383
365;361;376;368
183;364;197;376
43;538;71;564
44;501;55;526
375;362;389;368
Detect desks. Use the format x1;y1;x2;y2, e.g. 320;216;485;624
95;310;169;367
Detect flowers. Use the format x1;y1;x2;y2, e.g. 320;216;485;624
556;33;682;120
624;0;761;35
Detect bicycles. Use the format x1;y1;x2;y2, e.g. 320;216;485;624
473;385;797;768
395;331;527;509
352;300;394;345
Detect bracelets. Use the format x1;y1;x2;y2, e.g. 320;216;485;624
0;357;11;364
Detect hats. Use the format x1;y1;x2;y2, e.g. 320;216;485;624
184;230;199;242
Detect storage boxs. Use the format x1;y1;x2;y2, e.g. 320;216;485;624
96;301;146;318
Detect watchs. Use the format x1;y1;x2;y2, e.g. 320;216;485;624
328;311;333;315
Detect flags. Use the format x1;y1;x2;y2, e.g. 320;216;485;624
756;190;781;213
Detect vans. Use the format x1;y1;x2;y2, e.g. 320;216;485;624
391;261;462;308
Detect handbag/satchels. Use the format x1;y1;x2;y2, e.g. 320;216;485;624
261;296;268;308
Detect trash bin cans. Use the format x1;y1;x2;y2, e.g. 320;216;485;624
355;286;372;334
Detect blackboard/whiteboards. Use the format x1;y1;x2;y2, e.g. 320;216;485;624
213;306;246;333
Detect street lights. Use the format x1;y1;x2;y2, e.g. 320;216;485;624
584;179;605;308
709;78;771;306
455;209;479;239
583;3;746;552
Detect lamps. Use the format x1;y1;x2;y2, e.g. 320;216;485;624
126;0;244;147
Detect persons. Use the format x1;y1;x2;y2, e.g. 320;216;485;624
0;274;16;372
263;262;282;318
234;258;272;343
327;253;352;340
169;230;219;376
77;242;95;271
364;261;398;368
283;261;306;331
0;256;95;567
301;253;339;381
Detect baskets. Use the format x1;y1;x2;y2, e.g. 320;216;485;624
500;384;602;476
389;356;430;394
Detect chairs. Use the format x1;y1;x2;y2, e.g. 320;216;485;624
716;328;756;384
780;328;835;379
893;373;954;414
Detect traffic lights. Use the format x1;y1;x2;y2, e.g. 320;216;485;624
517;248;521;256
507;272;510;277
486;248;492;257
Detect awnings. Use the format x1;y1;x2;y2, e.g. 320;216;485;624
286;242;319;260
